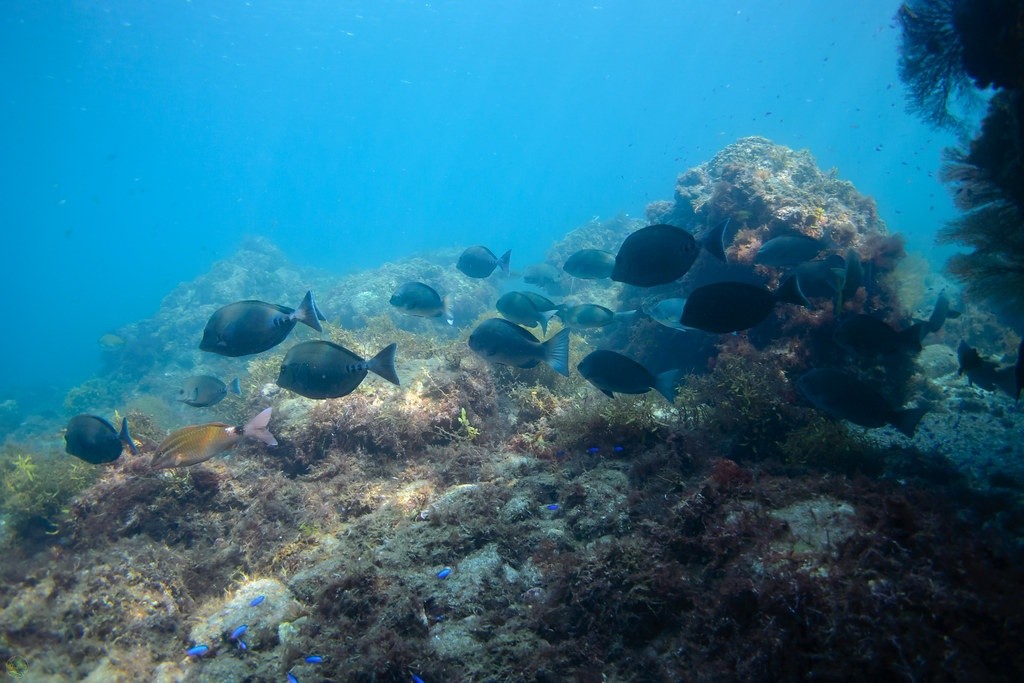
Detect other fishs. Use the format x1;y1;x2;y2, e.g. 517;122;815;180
62;1;1023;683
